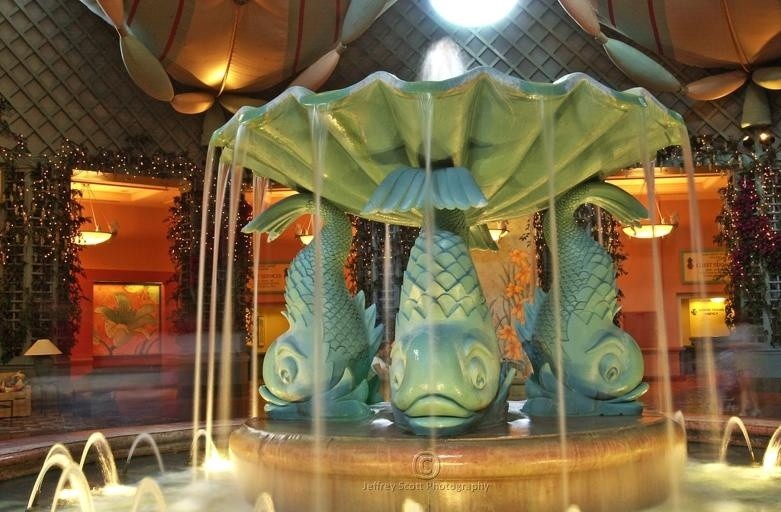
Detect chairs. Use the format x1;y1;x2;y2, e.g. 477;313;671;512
71;366;122;420
0;372;31;418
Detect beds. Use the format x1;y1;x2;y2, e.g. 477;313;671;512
29;380;61;416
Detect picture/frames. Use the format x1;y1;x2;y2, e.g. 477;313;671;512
679;248;726;285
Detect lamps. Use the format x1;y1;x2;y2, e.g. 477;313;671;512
69;184;119;247
23;338;62;379
621;180;680;240
